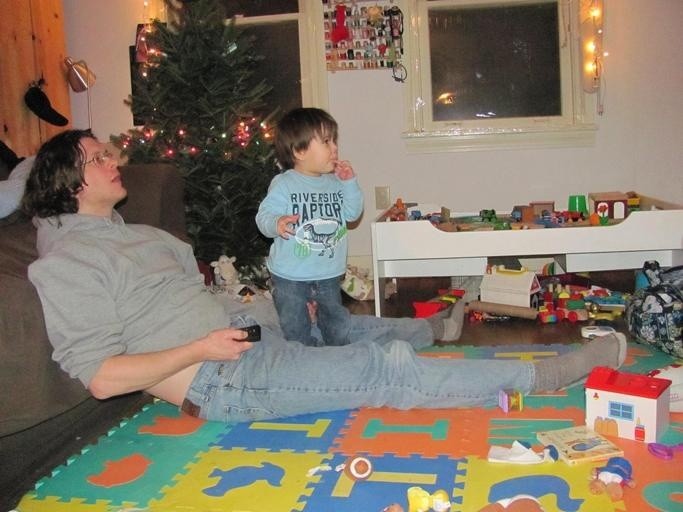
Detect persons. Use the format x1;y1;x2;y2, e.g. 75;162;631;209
25;128;628;426
254;107;364;348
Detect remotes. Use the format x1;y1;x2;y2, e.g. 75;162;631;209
233;325;261;341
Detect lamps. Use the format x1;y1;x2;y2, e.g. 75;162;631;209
64;58;97;131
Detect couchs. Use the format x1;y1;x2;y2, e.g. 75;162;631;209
0;161;184;436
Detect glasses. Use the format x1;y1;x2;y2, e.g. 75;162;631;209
81;153;119;168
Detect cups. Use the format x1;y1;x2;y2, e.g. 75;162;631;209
569;194;587;213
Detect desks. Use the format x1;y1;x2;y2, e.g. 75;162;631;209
372;196;681;319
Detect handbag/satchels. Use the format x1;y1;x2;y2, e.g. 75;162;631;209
624;282;682;358
